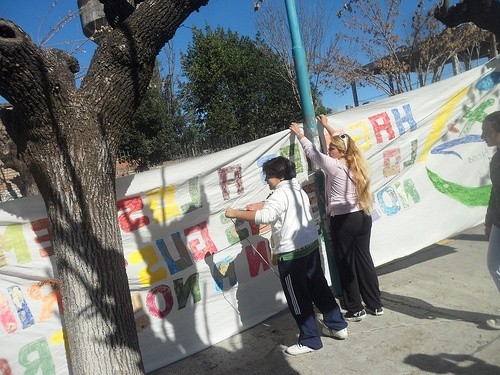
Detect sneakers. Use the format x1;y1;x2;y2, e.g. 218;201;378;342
486;318;500;329
365;306;383;314
343;309;366;321
286;344;314;355
323;325;347;339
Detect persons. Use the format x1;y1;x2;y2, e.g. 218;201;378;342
225;156;348;355
481;110;500;334
290;112;384;322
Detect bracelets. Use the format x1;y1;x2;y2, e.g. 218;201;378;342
297;131;302;137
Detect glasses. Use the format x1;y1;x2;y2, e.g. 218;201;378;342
335;134;347;150
